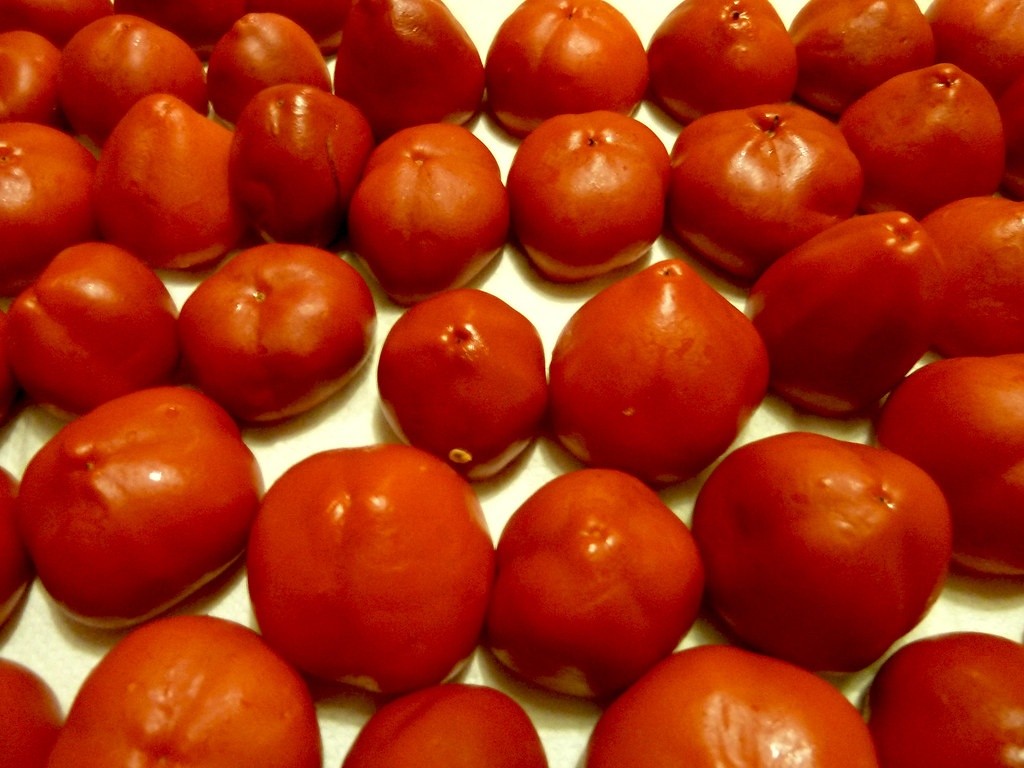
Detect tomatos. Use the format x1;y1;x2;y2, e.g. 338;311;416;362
0;0;1023;768
